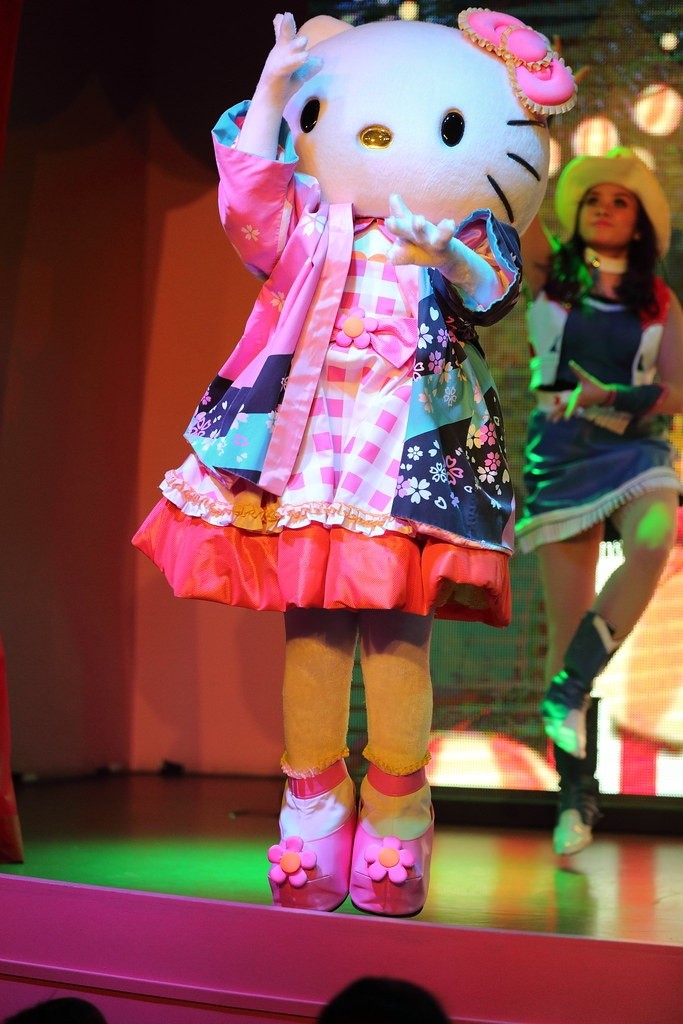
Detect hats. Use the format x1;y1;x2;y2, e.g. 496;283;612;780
554;146;671;261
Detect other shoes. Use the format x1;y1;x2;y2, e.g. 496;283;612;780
541;691;594;758
554;779;600;858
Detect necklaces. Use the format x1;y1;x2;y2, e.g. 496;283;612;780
583;244;629;274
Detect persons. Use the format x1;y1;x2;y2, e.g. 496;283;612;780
517;146;680;854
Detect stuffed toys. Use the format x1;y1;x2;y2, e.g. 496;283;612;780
130;6;584;919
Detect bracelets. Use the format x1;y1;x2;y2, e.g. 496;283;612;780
598;385;617;409
